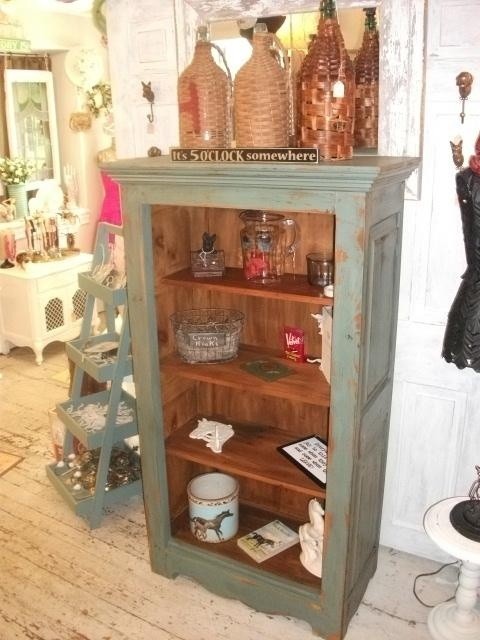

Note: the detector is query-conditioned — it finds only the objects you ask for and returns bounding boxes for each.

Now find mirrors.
[7,69,64,192]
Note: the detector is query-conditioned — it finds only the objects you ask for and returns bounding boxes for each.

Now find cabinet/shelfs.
[105,158,417,637]
[42,220,132,530]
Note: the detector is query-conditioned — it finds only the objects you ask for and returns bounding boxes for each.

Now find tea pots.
[236,212,299,286]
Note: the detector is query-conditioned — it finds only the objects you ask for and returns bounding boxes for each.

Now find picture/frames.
[278,435,339,488]
